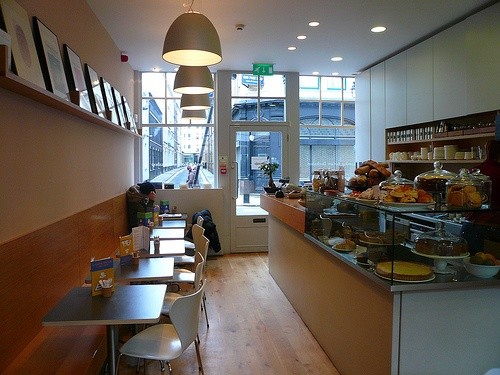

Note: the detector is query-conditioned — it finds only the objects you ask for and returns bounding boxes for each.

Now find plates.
[390,145,471,160]
[356,197,377,203]
[411,248,470,259]
[373,269,435,283]
[359,240,401,245]
[332,245,354,252]
[357,261,370,265]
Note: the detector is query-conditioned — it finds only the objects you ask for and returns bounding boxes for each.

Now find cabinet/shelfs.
[385,110,500,163]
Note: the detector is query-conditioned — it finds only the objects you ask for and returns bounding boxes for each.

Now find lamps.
[162,0,223,119]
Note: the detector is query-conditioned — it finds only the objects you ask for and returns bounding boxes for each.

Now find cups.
[103,286,112,297]
[132,257,139,264]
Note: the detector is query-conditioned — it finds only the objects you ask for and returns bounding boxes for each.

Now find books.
[131,225,150,251]
[145,207,159,225]
[91,256,115,296]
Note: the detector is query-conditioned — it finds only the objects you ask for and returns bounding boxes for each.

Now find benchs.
[0,194,130,375]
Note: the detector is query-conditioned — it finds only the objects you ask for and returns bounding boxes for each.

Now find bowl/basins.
[463,257,500,278]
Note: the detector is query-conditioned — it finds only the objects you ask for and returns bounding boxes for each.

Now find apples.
[470,252,498,266]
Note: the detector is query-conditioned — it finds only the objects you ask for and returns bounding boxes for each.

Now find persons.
[187,165,195,188]
[126,182,156,234]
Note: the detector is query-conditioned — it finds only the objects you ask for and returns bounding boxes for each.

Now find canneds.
[388,127,435,143]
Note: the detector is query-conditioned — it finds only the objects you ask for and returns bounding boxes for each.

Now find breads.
[348,159,391,186]
[335,242,356,250]
[380,185,433,204]
[357,188,378,200]
[447,185,483,208]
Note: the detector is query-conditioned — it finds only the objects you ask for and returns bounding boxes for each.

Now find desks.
[149,228,184,239]
[155,220,186,228]
[150,240,186,255]
[40,284,167,375]
[163,212,188,220]
[85,257,175,284]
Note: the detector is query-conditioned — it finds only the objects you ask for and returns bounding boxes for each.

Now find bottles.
[446,168,491,208]
[435,119,496,134]
[160,199,169,214]
[413,161,456,205]
[312,171,321,193]
[378,170,414,200]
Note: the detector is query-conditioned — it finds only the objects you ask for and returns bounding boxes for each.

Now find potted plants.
[260,163,280,193]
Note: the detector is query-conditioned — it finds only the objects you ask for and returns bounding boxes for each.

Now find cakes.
[414,232,468,256]
[359,231,405,244]
[375,262,432,280]
[415,175,456,191]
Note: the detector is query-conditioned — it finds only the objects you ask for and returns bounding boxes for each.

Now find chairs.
[116,211,210,375]
